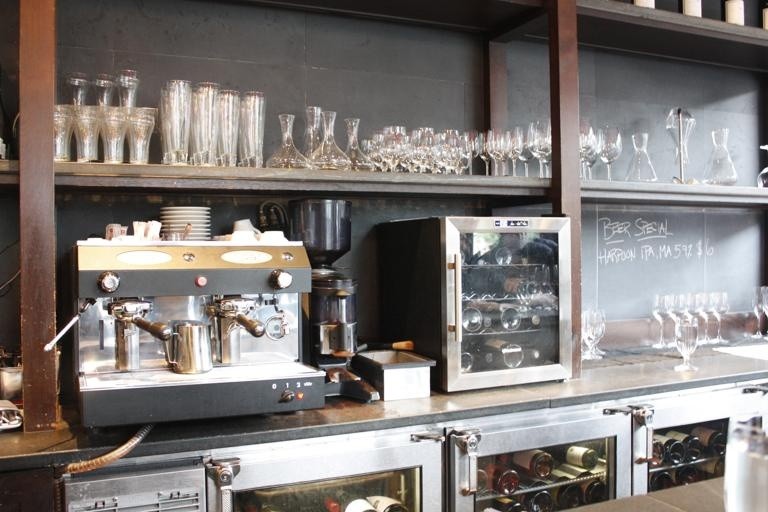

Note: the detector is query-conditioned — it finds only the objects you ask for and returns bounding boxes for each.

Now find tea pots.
[162,318,214,377]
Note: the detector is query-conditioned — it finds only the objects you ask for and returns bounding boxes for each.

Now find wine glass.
[579,306,609,362]
[362,120,623,182]
[651,286,767,372]
[756,145,768,187]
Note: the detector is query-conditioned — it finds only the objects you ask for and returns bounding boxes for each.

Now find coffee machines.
[288,198,360,401]
[71,243,324,433]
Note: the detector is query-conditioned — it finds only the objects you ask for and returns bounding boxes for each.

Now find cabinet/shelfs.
[1,1,768,437]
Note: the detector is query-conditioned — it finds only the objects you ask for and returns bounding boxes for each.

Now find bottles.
[663,107,699,183]
[245,488,414,512]
[623,131,659,184]
[460,236,558,374]
[265,104,372,170]
[473,430,728,510]
[704,128,735,185]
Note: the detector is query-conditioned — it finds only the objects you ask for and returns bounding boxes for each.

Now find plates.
[160,205,212,241]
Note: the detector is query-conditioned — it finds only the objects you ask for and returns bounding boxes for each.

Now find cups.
[53,71,157,165]
[678,0,702,17]
[105,223,128,241]
[632,0,655,9]
[724,0,744,25]
[158,79,264,167]
[723,412,766,512]
[230,220,287,242]
